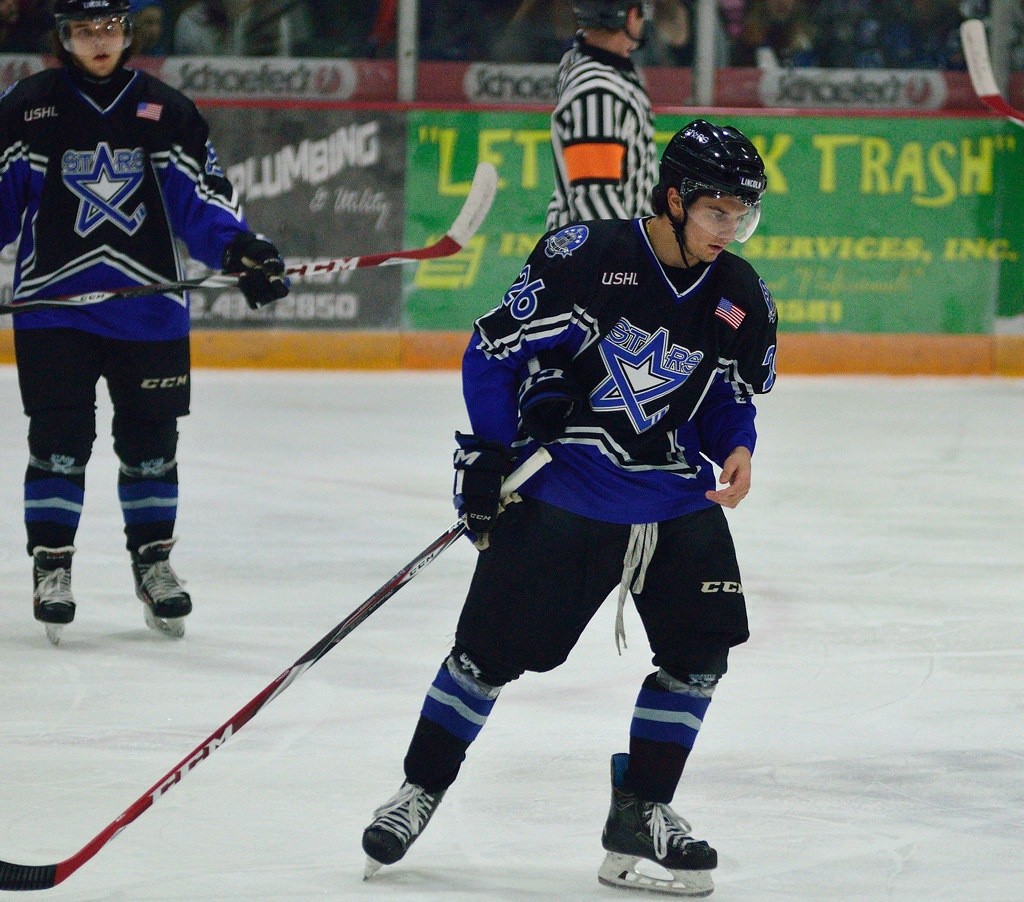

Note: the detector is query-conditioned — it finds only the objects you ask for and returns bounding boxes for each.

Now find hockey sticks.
[1,161,499,317]
[0,448,553,892]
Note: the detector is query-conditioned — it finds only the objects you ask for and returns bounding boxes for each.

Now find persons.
[0,0,1024,70]
[0,0,292,646]
[363,118,779,898]
[545,0,658,233]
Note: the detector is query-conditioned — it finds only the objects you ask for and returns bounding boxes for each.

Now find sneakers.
[598,754,717,896]
[361,778,447,881]
[33,546,76,647]
[129,537,192,638]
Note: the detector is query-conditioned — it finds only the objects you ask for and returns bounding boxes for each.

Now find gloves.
[223,233,291,309]
[451,430,525,559]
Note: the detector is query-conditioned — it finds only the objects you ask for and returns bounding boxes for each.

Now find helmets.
[50,0,131,23]
[573,0,645,32]
[661,118,769,203]
